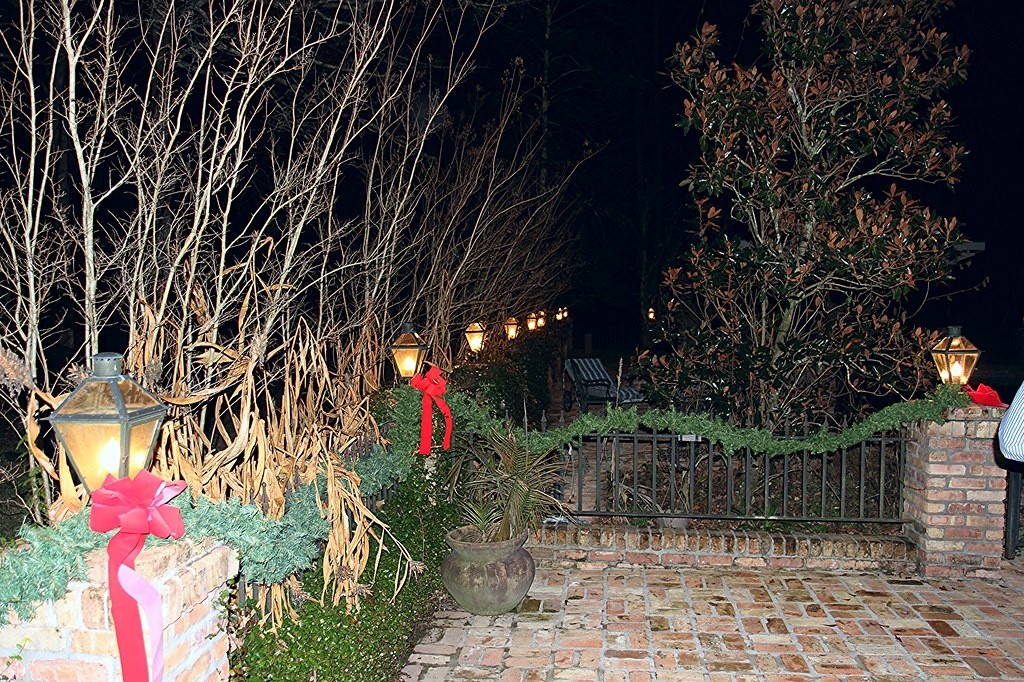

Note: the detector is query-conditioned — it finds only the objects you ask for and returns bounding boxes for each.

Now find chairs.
[562,358,646,414]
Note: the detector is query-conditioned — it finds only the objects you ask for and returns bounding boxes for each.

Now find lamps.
[39,353,174,495]
[930,326,986,386]
[526,306,569,331]
[464,322,488,359]
[388,323,430,385]
[504,316,521,342]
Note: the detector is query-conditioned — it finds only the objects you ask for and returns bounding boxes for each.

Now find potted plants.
[442,428,572,616]
